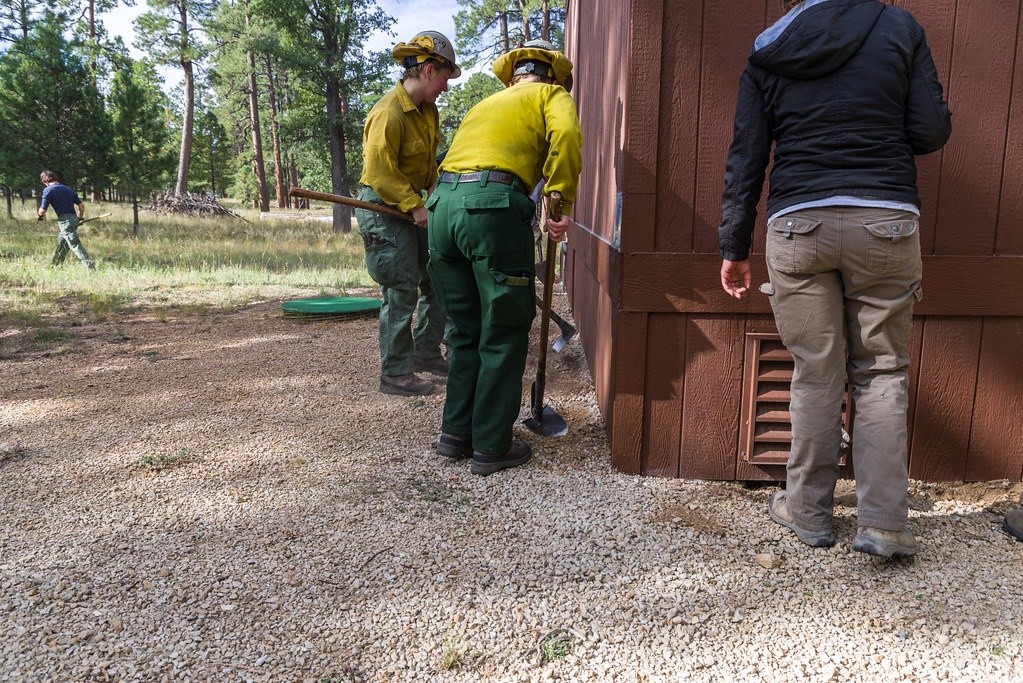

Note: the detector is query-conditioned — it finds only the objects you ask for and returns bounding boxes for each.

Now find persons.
[718,0,954,561]
[355,30,462,394]
[426,42,582,475]
[36,170,95,270]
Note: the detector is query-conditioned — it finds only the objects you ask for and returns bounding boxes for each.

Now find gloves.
[37,214,43,221]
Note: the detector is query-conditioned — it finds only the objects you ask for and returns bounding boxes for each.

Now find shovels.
[532,212,556,286]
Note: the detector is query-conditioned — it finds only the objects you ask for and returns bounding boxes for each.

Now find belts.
[440,172,528,195]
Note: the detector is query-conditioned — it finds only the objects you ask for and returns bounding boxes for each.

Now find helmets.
[494,39,573,93]
[393,30,461,78]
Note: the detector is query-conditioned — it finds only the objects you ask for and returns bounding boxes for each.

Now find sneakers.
[379,372,436,396]
[436,431,472,458]
[852,526,917,557]
[769,489,835,547]
[471,439,530,476]
[414,355,449,376]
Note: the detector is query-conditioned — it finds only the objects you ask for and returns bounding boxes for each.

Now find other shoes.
[1004,510,1023,540]
